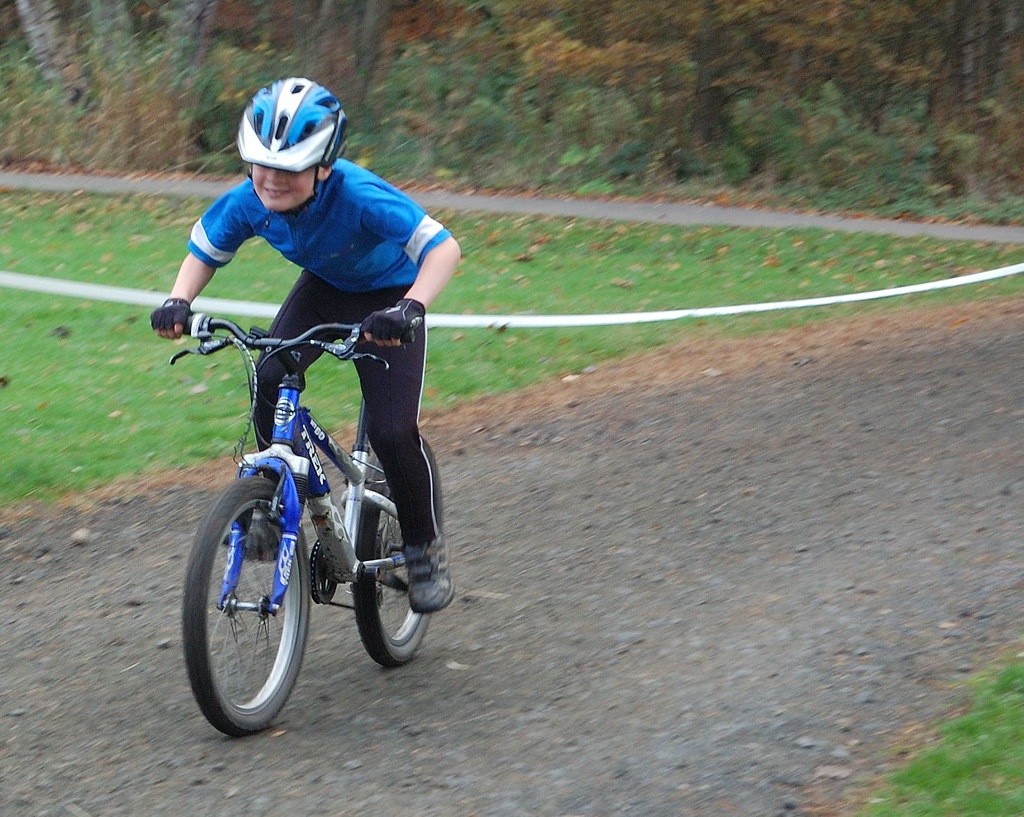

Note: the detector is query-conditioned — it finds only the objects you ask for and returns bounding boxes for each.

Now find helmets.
[237,78,347,173]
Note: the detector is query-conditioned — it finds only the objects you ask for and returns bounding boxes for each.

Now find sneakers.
[224,509,282,562]
[405,534,455,613]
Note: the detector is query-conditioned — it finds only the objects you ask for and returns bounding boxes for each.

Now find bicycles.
[167,311,433,741]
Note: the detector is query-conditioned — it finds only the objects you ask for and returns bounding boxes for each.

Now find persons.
[150,77,463,614]
